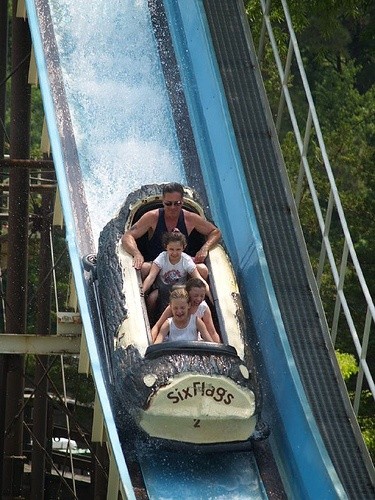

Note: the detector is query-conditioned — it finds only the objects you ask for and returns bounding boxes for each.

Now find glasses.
[162,200,183,208]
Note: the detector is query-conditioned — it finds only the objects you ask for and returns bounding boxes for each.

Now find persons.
[149,278,222,343]
[119,181,221,284]
[152,283,214,347]
[138,228,214,304]
